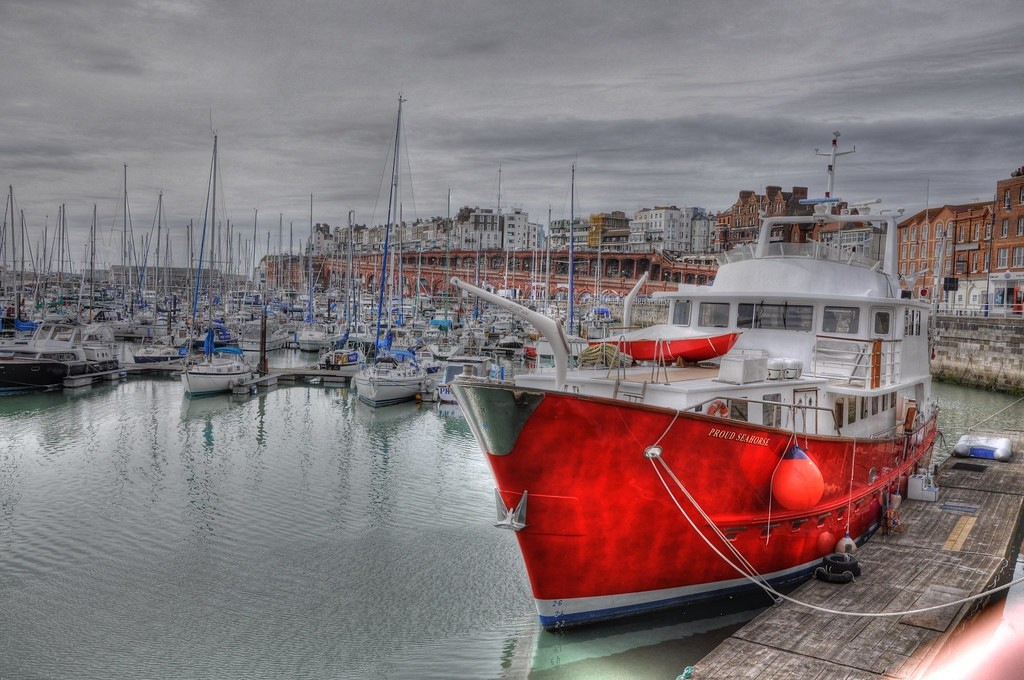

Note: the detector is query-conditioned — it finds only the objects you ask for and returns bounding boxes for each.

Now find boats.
[451,129,942,633]
[586,323,744,363]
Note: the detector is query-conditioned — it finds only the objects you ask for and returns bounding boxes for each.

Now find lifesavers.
[707,400,729,418]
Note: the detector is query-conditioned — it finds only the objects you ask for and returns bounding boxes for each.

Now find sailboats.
[0,92,620,409]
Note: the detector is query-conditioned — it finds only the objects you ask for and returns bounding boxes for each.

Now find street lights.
[983,193,1013,317]
[943,213,958,278]
